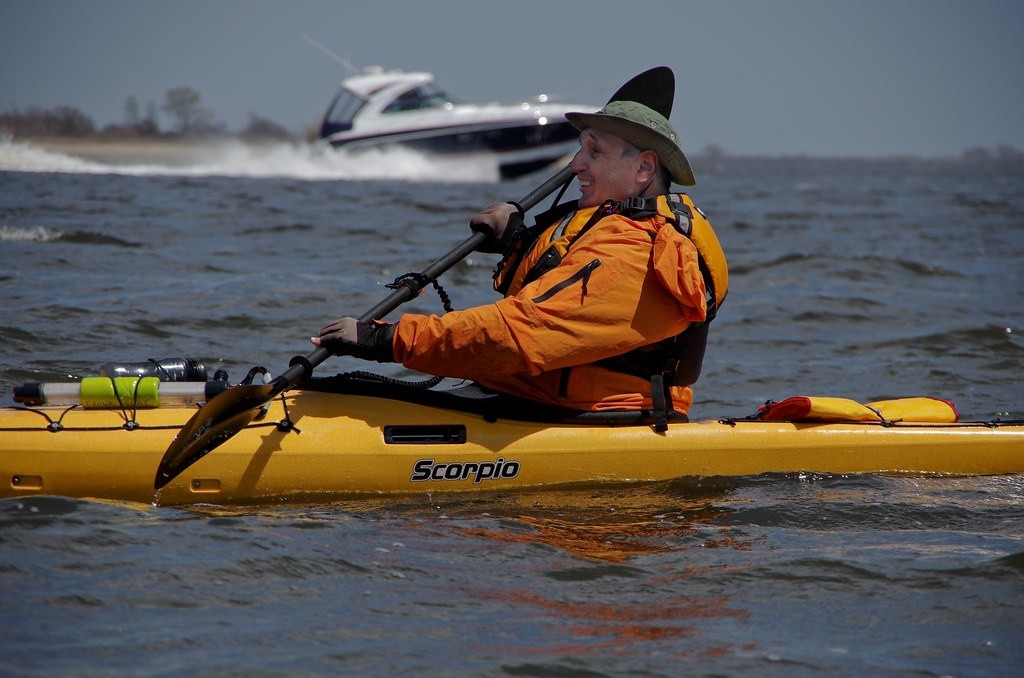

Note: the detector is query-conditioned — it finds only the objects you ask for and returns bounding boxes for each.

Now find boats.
[0,375,1024,497]
[309,69,602,182]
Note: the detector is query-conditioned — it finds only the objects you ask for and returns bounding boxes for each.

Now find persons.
[310,101,729,425]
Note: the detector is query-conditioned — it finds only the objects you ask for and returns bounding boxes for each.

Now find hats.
[564,66,697,186]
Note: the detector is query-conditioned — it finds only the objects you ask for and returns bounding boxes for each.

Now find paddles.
[155,161,579,494]
